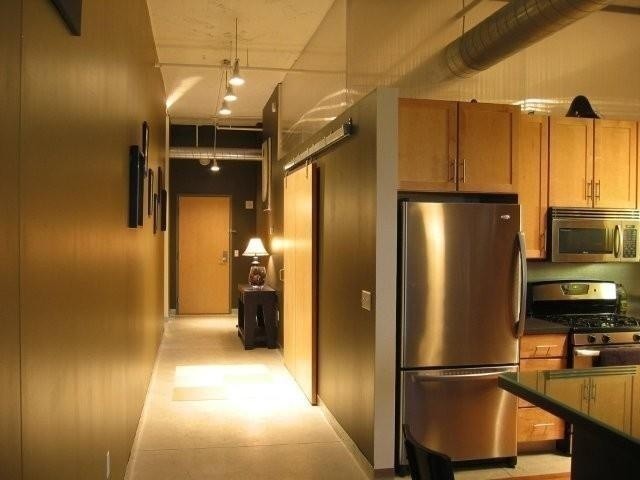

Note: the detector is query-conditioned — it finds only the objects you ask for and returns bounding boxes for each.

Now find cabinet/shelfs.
[516,332,568,455]
[548,116,636,212]
[396,96,521,194]
[237,283,277,351]
[517,112,549,261]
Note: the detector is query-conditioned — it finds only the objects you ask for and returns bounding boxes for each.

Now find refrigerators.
[397,189,529,476]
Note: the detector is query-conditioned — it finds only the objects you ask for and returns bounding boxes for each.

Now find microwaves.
[550,217,639,263]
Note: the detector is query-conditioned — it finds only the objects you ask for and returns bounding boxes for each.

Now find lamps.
[210,120,219,172]
[216,17,245,116]
[241,238,269,288]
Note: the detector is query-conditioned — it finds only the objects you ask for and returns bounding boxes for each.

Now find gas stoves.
[538,311,640,345]
